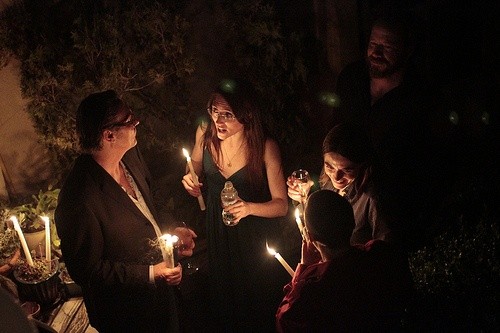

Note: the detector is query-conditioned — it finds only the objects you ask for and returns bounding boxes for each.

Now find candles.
[155,233,180,269]
[265,242,294,280]
[290,208,311,241]
[179,148,211,211]
[40,213,51,263]
[11,214,38,267]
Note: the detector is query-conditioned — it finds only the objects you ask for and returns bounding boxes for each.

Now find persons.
[332,17,452,247]
[275,191,424,333]
[54,88,197,333]
[287,123,400,252]
[182,87,301,310]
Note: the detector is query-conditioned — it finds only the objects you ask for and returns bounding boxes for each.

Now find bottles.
[220,180,240,227]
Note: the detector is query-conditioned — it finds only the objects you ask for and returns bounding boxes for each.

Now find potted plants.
[59,266,84,296]
[0,227,20,278]
[11,204,48,258]
[13,252,62,309]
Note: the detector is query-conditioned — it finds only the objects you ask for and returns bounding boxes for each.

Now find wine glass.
[168,221,201,275]
[291,169,311,227]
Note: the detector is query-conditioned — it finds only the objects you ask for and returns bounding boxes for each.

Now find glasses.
[103,112,138,129]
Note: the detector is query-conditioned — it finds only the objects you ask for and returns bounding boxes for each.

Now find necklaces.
[223,141,243,167]
